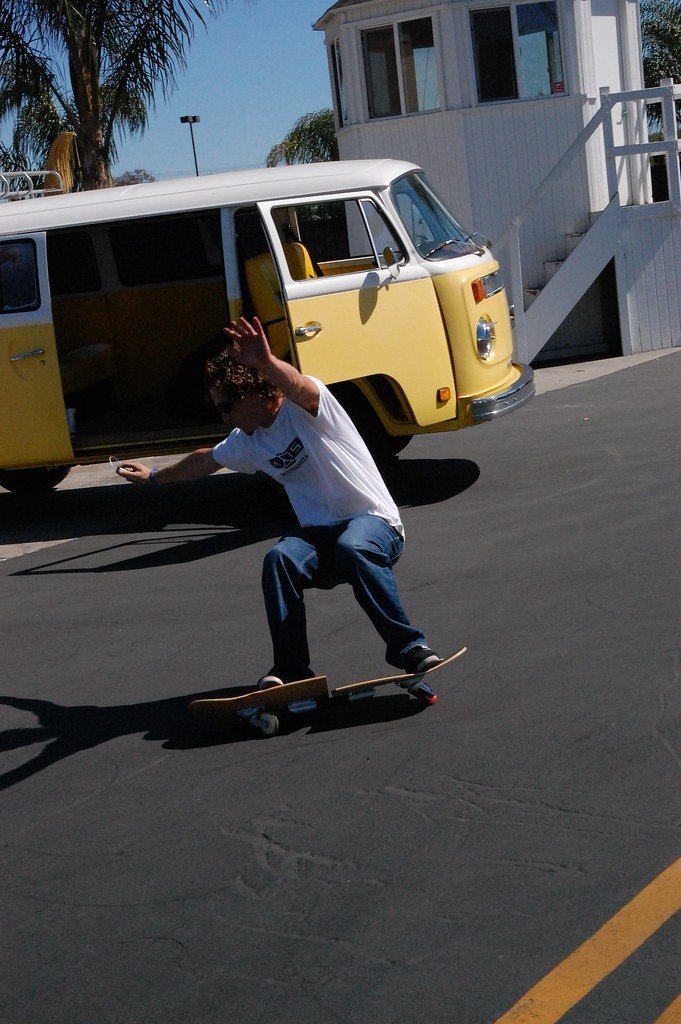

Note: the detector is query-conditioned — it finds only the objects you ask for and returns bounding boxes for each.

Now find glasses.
[216,397,236,414]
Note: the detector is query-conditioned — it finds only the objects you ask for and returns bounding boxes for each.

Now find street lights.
[179,114,200,176]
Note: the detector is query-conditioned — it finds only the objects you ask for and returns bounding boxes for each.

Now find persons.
[116,316,442,689]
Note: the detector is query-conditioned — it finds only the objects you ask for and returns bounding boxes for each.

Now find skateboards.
[186,644,468,737]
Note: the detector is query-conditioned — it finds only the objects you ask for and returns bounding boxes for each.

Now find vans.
[1,166,537,499]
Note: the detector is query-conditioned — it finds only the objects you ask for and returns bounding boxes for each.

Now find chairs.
[58,344,115,425]
[245,243,319,361]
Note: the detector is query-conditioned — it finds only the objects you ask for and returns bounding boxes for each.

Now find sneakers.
[386,646,440,673]
[261,666,303,688]
